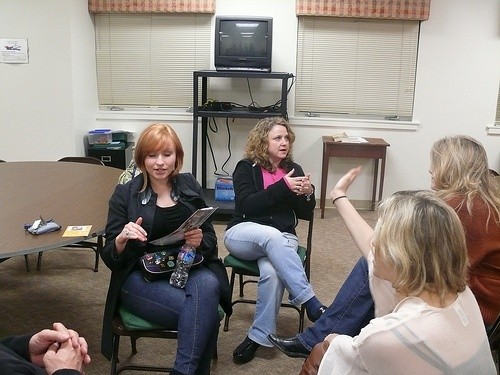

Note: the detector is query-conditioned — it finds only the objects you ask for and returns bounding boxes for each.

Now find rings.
[126,232,130,236]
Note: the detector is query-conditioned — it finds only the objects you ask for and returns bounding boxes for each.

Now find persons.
[297,165,497,375]
[268,136,500,358]
[224,116,329,365]
[101,124,233,370]
[0,323,92,375]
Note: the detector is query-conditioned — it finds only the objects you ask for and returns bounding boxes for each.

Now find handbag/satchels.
[140,246,204,282]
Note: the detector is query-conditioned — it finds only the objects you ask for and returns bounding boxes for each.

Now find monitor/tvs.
[214,16,273,73]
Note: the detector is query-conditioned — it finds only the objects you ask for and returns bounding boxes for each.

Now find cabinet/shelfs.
[192,71,293,225]
[88,143,134,171]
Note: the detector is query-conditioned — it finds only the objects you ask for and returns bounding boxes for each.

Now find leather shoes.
[233,336,260,364]
[268,333,311,357]
[306,305,327,322]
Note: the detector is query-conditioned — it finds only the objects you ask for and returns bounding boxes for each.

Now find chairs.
[0,157,106,272]
[111,305,225,375]
[220,184,315,333]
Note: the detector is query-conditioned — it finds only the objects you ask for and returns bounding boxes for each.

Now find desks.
[0,161,125,257]
[320,136,390,219]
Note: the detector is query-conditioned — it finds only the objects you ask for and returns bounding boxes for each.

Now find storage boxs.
[215,176,235,202]
[89,130,110,145]
[113,133,127,142]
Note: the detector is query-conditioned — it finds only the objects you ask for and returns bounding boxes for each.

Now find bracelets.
[308,185,314,196]
[332,196,347,205]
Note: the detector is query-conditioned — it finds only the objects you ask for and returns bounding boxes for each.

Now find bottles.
[169,245,196,289]
